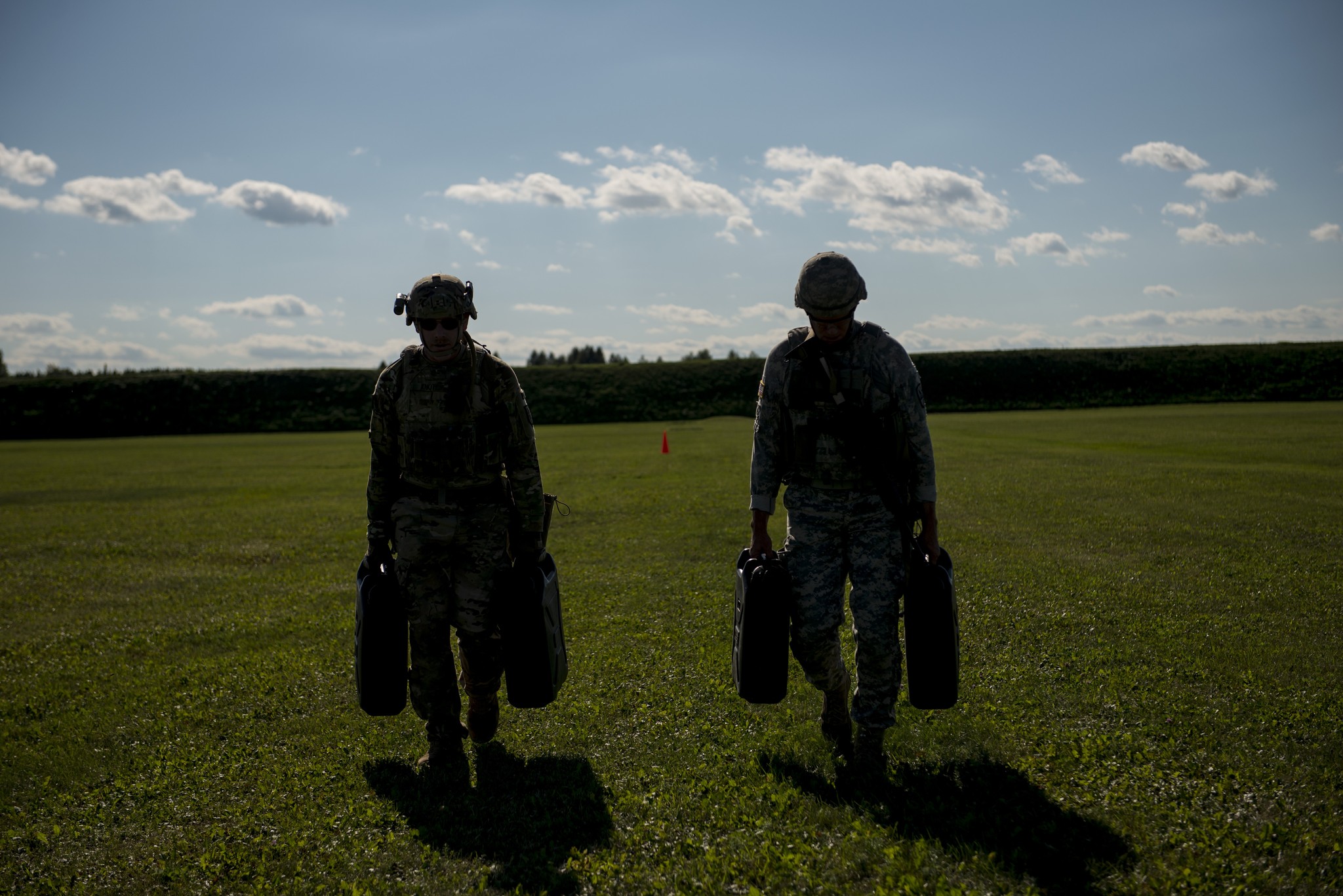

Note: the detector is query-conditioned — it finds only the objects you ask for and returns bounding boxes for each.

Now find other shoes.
[418,740,463,769]
[854,726,884,768]
[820,672,853,744]
[467,693,500,743]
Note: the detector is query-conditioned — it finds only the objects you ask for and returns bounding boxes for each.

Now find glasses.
[417,315,460,331]
[809,310,854,327]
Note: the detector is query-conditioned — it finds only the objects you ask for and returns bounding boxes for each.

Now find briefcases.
[730,549,793,704]
[355,558,407,716]
[504,552,569,708]
[904,547,960,709]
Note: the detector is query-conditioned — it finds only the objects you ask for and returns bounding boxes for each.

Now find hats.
[795,252,867,316]
[393,273,478,326]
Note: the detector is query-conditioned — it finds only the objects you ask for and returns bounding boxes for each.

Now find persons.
[731,252,945,791]
[362,272,553,782]
